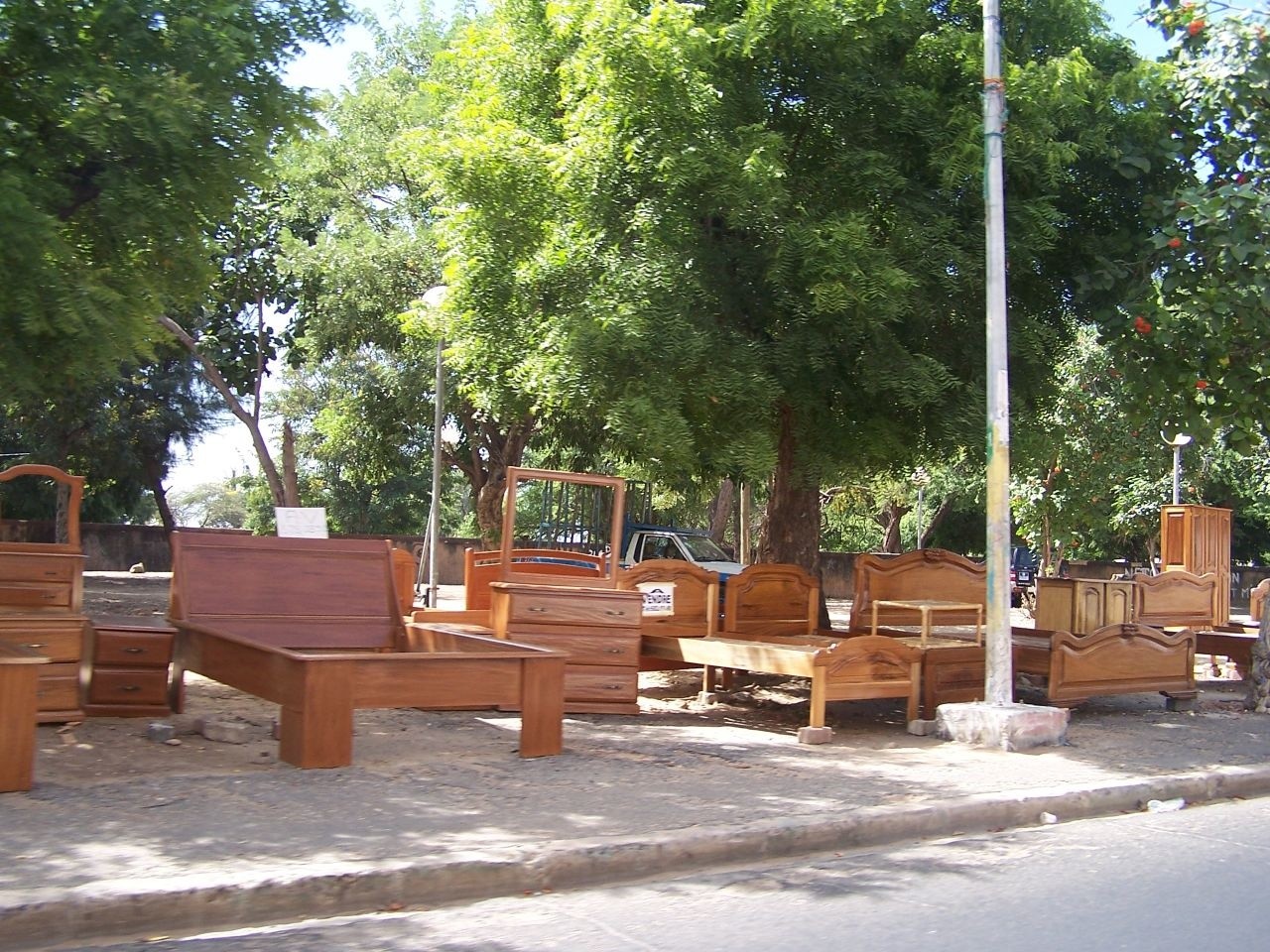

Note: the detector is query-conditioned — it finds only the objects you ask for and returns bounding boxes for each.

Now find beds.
[164,527,574,769]
[720,564,991,726]
[409,544,605,614]
[846,546,1197,711]
[1125,571,1257,684]
[614,559,929,733]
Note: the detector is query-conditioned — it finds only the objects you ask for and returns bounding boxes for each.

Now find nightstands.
[80,613,178,720]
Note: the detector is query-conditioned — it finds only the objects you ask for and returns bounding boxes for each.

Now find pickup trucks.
[524,525,753,605]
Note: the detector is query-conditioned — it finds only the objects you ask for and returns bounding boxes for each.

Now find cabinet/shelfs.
[1033,573,1137,640]
[487,465,645,716]
[0,464,88,729]
[1159,502,1234,631]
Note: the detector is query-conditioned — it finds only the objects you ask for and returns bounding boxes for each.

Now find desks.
[0,638,54,793]
[870,596,984,650]
